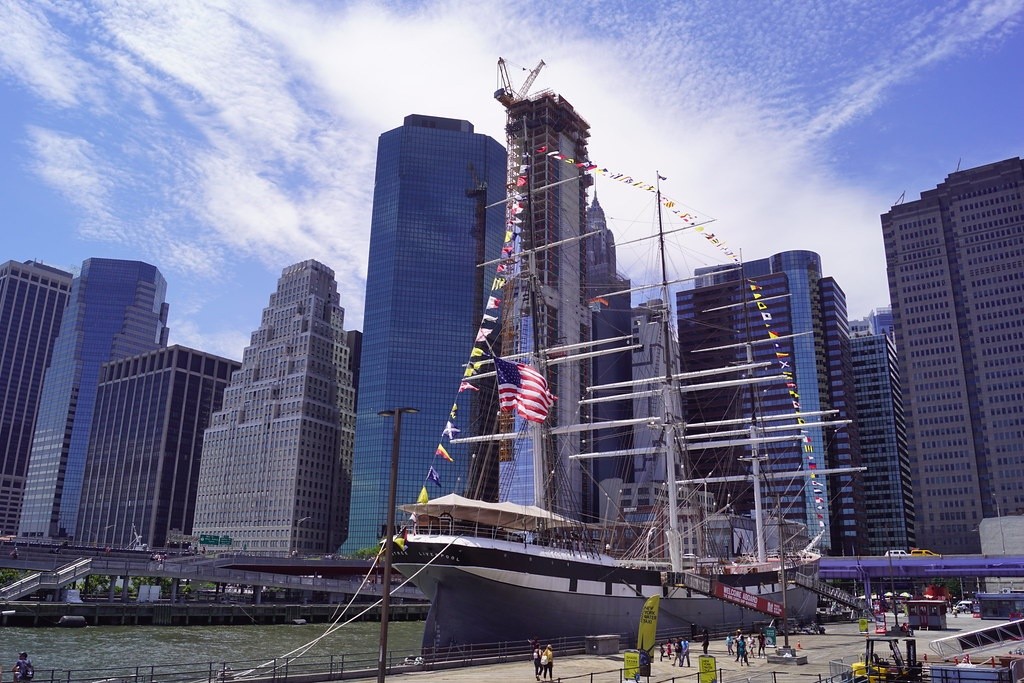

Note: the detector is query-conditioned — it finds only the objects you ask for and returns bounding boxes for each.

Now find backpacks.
[22,659,34,681]
[541,656,548,665]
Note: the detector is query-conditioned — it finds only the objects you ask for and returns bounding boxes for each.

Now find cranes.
[492,56,548,110]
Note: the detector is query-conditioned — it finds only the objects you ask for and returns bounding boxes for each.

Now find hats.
[547,645,552,649]
[736,629,742,633]
[18,652,27,658]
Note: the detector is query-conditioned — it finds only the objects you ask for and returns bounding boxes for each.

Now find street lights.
[295,517,313,557]
[376,407,421,683]
[993,493,1005,554]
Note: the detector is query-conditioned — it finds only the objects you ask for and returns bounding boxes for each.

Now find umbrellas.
[884,591,893,596]
[900,591,913,597]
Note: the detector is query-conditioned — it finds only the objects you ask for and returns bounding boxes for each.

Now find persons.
[680,636,691,667]
[666,639,674,660]
[542,645,555,681]
[11,652,33,683]
[528,636,538,661]
[659,643,668,662]
[735,629,750,666]
[671,637,681,666]
[757,631,766,659]
[702,628,709,655]
[747,633,756,657]
[725,631,735,655]
[533,643,543,681]
[953,605,957,617]
[962,603,966,613]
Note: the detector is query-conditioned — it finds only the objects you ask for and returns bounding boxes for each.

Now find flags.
[393,166,530,549]
[537,144,827,530]
[493,355,559,423]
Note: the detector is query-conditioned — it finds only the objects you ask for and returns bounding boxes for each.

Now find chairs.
[873,654,889,666]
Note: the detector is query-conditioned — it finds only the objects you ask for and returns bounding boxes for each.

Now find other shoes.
[536,675,540,681]
[672,663,676,666]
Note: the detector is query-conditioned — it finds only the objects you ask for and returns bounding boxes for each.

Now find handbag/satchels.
[751,643,756,648]
[534,653,538,658]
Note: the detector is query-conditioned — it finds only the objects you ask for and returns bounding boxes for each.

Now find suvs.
[885,550,911,557]
[910,550,940,556]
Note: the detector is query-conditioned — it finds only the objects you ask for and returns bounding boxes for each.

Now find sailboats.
[384,105,869,676]
